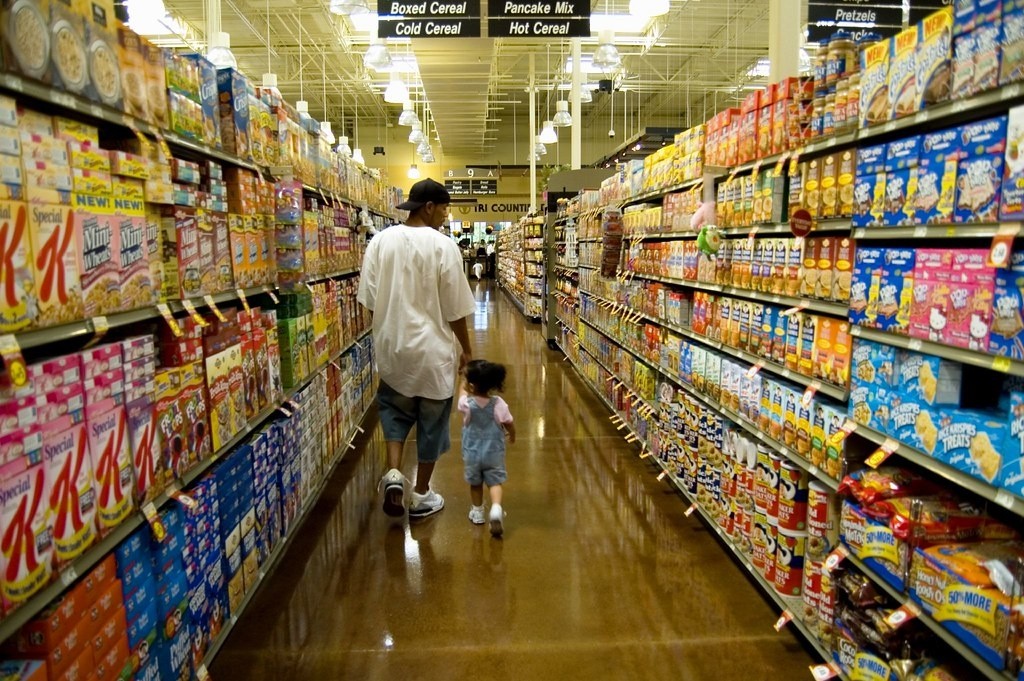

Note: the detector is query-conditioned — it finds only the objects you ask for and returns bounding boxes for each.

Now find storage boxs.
[580,0,1023,681]
[0,0,381,680]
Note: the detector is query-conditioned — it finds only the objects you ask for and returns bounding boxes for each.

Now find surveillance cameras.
[608,130,615,138]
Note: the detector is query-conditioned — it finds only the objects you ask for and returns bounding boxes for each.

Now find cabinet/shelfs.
[0,75,408,681]
[495,222,543,324]
[548,79,1023,681]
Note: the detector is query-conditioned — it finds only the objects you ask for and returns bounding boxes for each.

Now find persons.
[356,178,477,519]
[457,359,516,536]
[446,231,497,281]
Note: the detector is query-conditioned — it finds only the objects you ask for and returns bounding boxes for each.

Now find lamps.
[120,0,670,176]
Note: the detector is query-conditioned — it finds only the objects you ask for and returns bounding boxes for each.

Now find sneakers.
[378,469,410,517]
[469,508,486,524]
[409,485,444,518]
[488,506,507,535]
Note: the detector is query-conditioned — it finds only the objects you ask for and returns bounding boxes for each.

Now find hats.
[396,178,451,210]
